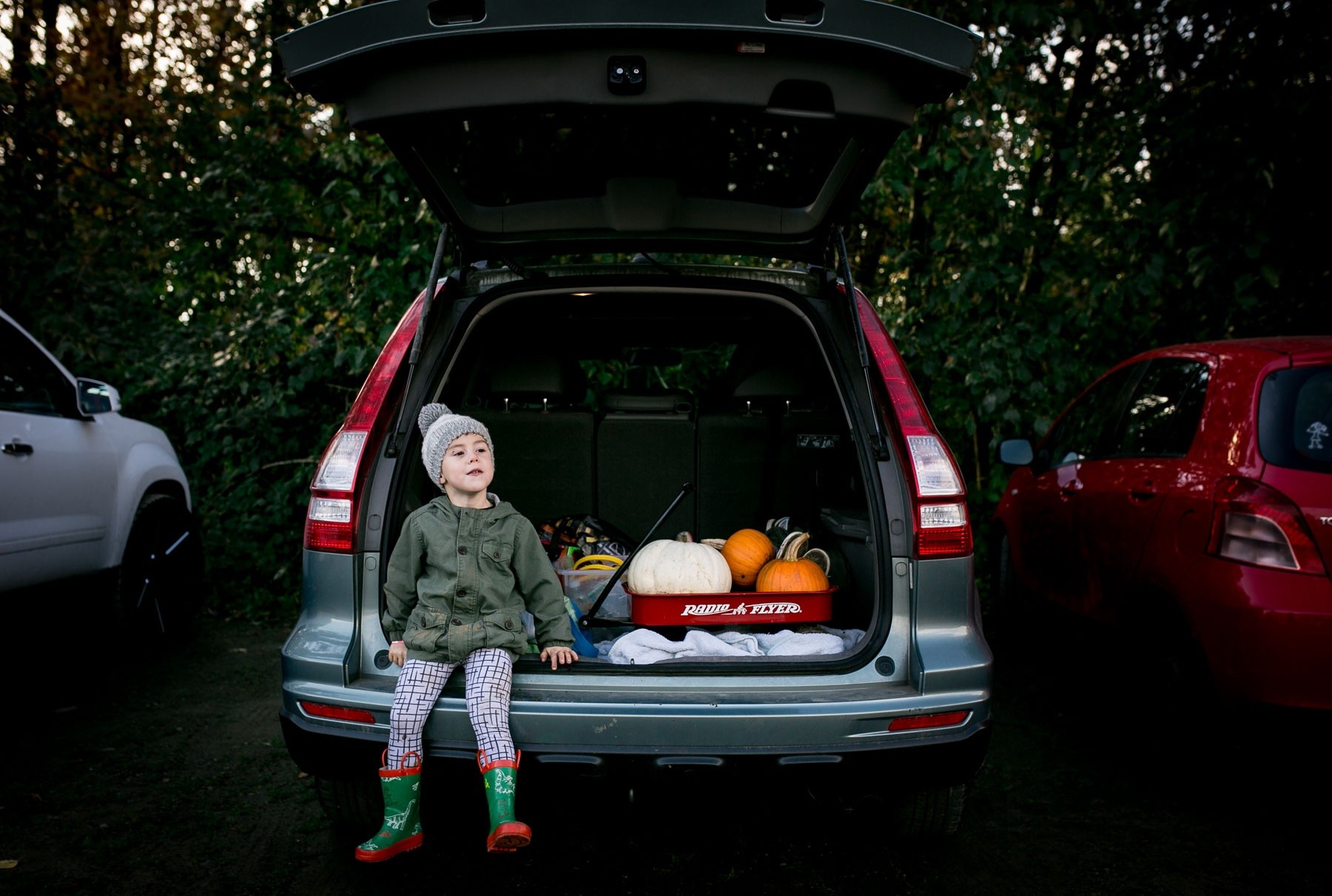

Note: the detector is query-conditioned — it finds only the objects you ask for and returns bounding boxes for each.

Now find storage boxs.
[553,560,632,623]
[625,584,835,626]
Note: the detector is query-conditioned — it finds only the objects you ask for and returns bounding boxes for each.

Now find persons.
[355,403,579,859]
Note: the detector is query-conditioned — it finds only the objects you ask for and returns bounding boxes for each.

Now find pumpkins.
[628,529,830,592]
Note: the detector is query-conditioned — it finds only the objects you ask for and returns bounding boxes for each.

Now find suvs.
[271,0,990,834]
[0,310,199,643]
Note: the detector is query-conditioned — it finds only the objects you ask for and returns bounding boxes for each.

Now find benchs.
[462,365,814,552]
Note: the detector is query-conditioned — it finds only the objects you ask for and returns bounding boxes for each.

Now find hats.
[418,404,494,492]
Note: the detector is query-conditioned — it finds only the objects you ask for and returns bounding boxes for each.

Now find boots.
[356,749,422,862]
[477,750,532,851]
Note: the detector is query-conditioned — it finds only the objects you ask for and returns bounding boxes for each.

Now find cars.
[994,333,1332,791]
